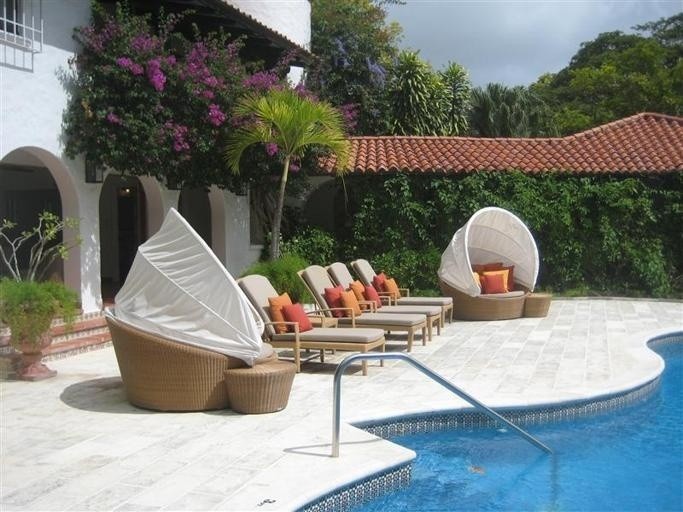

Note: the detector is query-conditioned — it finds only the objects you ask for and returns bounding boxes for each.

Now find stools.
[524,293,552,318]
[223,359,297,413]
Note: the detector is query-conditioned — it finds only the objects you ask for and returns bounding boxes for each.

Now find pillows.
[473,262,515,294]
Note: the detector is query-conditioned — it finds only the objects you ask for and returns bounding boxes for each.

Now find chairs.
[236,257,455,375]
[102,207,265,412]
[436,205,539,321]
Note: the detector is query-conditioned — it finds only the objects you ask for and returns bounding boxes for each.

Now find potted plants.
[0,212,84,382]
